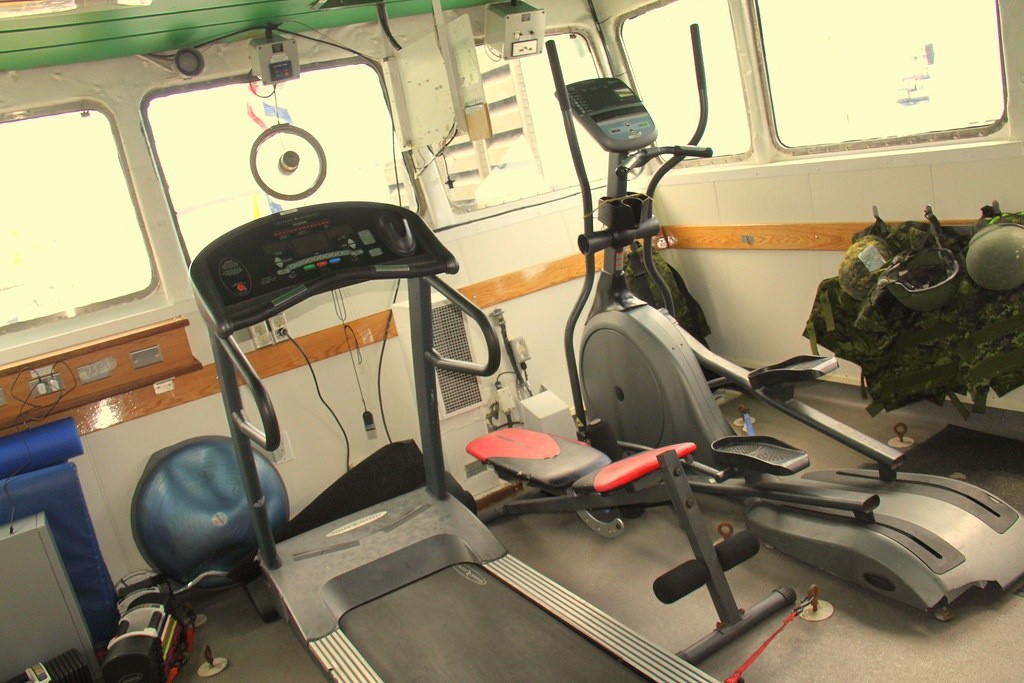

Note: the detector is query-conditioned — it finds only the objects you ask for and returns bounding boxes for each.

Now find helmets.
[965,222,1024,291]
[878,246,959,312]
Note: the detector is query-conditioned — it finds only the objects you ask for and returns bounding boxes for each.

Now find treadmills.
[186,199,723,683]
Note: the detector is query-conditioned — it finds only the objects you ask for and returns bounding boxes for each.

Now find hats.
[838,234,896,303]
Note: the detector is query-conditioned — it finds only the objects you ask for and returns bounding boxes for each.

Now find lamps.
[172,48,204,80]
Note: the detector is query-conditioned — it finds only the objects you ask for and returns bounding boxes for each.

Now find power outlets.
[248,313,291,350]
[28,372,66,399]
